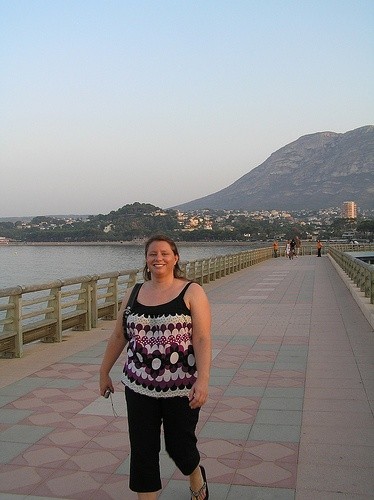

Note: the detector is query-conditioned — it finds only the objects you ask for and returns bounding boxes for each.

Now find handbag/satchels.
[122,282,143,340]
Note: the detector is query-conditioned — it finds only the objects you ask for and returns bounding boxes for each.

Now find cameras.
[104,388,111,398]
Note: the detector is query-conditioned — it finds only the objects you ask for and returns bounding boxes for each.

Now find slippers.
[189,465,209,500]
